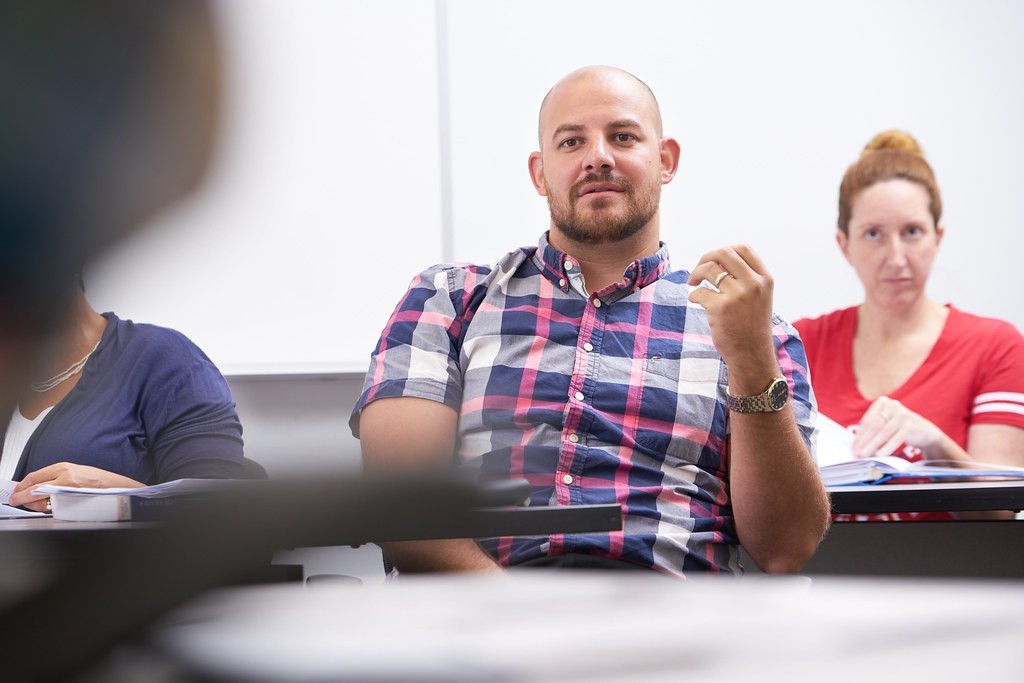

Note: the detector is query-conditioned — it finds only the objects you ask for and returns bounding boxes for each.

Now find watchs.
[725,375,790,414]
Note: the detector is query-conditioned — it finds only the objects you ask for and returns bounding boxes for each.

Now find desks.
[0,502,622,554]
[737,480,1024,580]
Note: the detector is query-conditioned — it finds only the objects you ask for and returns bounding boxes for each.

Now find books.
[817,456,1024,486]
[50,494,239,521]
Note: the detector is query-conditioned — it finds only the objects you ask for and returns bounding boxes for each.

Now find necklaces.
[27,338,100,392]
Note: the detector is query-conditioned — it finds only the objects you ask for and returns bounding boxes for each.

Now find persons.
[0,263,247,514]
[350,66,830,576]
[790,130,1023,481]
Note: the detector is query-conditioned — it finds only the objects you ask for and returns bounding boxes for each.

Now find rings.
[879,411,888,421]
[715,271,729,288]
[47,498,52,510]
